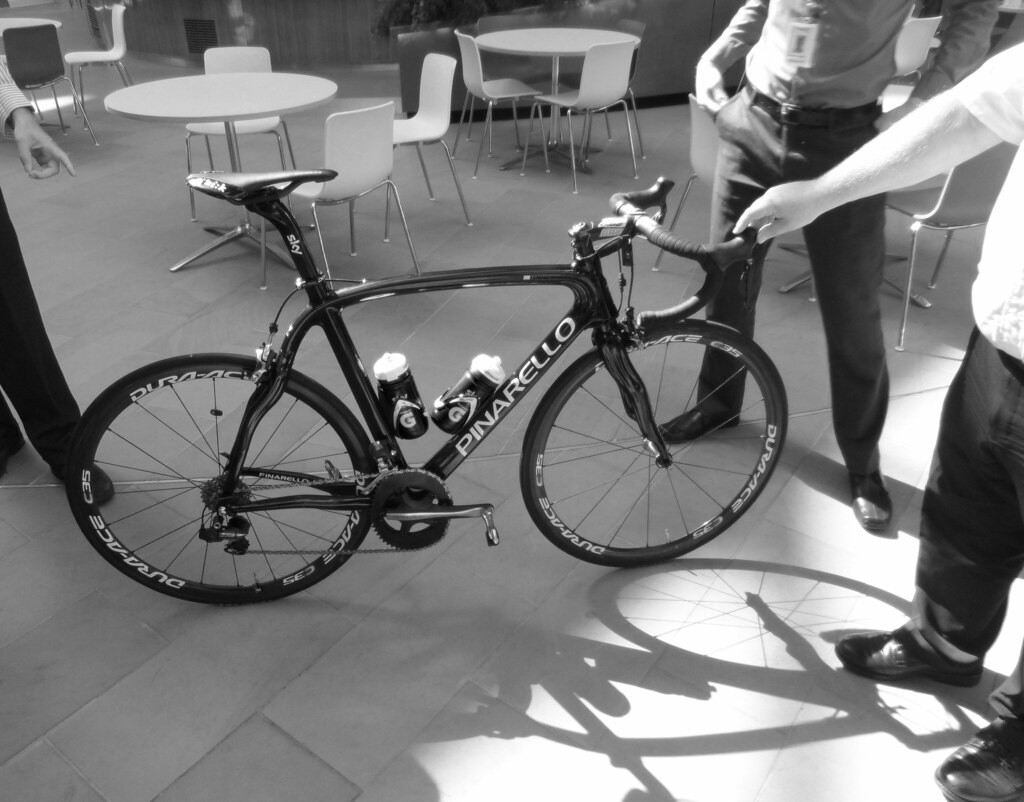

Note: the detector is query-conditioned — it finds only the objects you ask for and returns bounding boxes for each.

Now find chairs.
[261,101,421,312]
[64,4,134,131]
[520,19,647,195]
[354,53,473,243]
[652,93,768,273]
[883,141,1019,352]
[4,25,100,147]
[185,47,297,221]
[454,28,551,179]
[891,15,943,84]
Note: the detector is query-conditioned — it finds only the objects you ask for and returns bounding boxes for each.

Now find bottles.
[431,354,505,435]
[373,352,429,440]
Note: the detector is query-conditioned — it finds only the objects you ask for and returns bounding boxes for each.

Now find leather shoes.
[52,461,114,506]
[850,469,892,530]
[935,716,1024,802]
[834,624,984,688]
[658,408,741,444]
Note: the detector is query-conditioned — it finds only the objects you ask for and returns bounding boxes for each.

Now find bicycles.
[66,167,792,604]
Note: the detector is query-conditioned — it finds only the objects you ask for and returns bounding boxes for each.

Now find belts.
[746,79,882,128]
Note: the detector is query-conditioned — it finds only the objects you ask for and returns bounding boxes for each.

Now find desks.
[0,18,62,36]
[476,25,640,174]
[775,84,932,308]
[105,72,338,273]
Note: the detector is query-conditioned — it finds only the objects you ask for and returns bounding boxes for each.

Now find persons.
[0,58,115,506]
[732,40,1024,802]
[656,0,1001,530]
